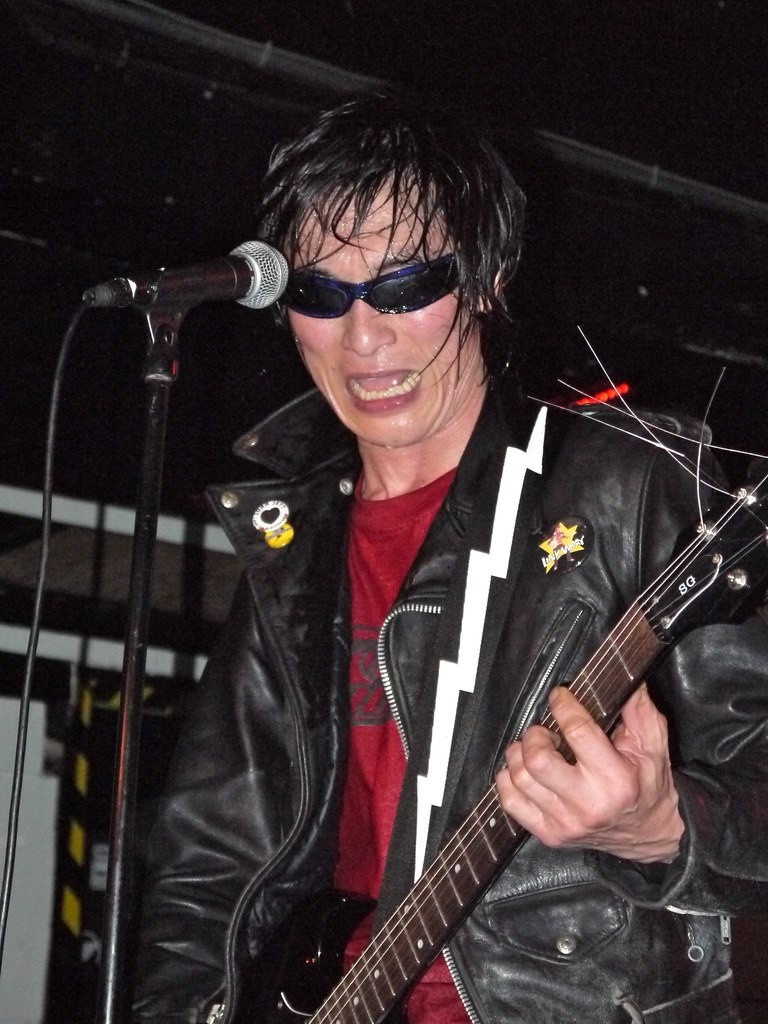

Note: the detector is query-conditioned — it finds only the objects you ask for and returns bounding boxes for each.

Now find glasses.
[278,252,461,320]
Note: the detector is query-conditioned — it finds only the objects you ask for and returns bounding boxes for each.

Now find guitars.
[207,468,767,1023]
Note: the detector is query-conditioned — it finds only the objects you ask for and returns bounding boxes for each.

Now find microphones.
[83,239,292,312]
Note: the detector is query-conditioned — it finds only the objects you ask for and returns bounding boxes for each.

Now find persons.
[110,93,768,1023]
